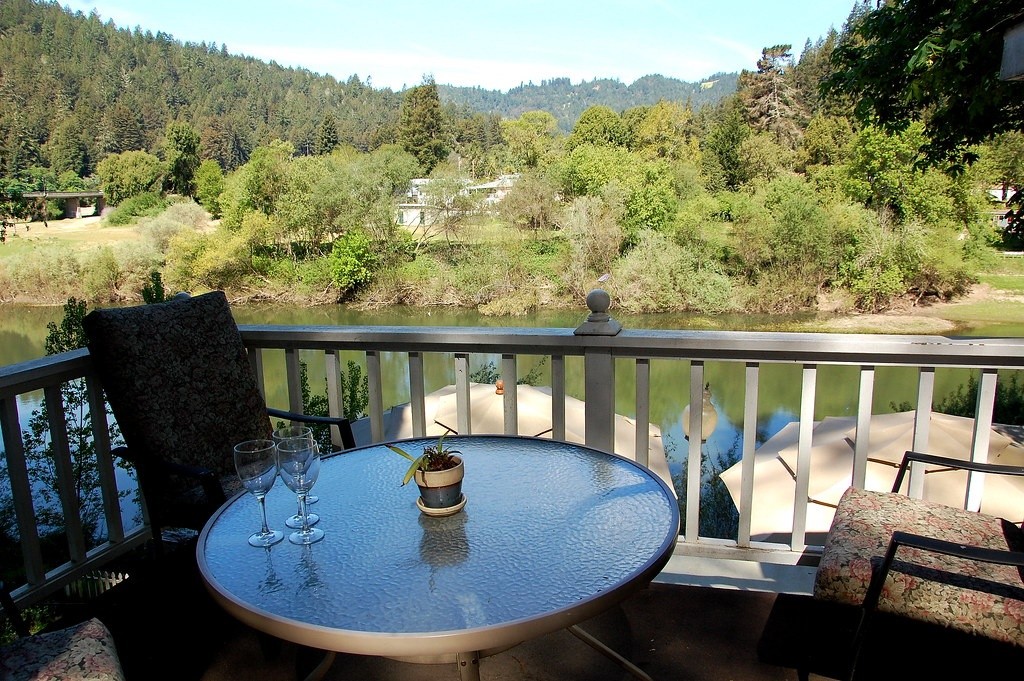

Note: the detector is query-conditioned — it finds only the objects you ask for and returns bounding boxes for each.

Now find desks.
[204,431,679,681]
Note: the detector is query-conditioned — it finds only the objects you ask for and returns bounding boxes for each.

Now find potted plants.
[382,428,467,517]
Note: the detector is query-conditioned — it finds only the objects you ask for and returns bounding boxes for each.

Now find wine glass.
[281,434,319,505]
[234,440,284,547]
[272,427,319,528]
[277,437,325,545]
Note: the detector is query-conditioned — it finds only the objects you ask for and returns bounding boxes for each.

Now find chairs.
[79,289,357,602]
[796,417,1024,681]
